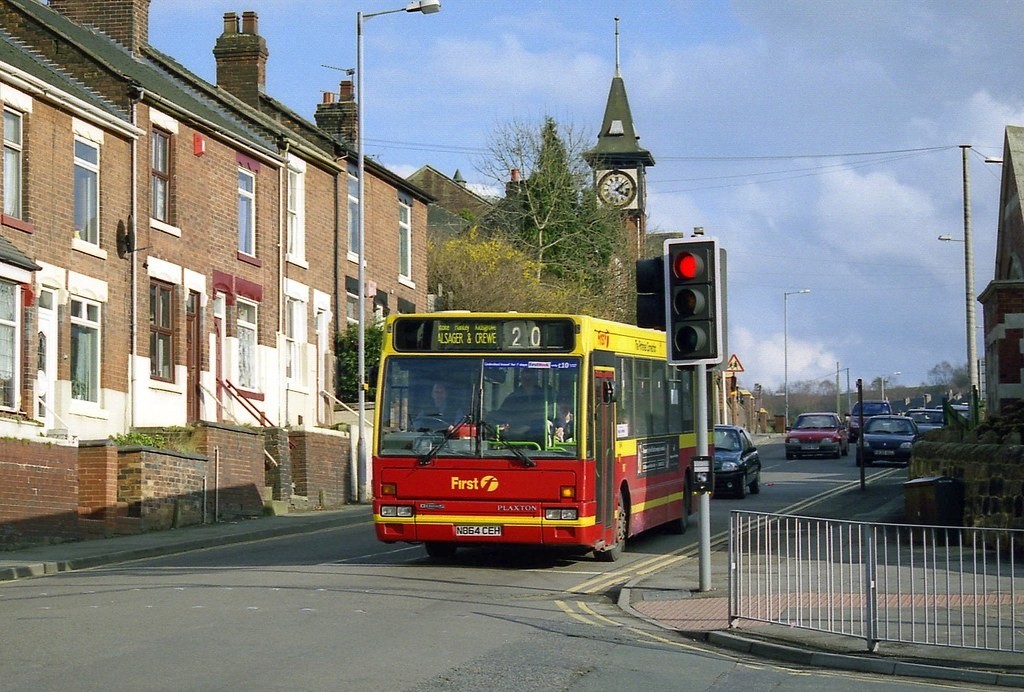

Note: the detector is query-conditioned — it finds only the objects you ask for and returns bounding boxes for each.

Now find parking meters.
[689,456,716,496]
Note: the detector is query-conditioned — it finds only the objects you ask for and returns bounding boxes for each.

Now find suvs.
[845,400,894,443]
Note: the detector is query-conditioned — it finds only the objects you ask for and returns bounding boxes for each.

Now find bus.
[372,308,716,562]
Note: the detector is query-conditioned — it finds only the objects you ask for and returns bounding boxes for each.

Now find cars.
[785,411,849,458]
[856,415,920,468]
[936,405,969,425]
[712,426,763,501]
[903,408,945,434]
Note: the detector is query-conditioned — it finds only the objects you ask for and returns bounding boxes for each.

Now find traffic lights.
[636,257,666,331]
[665,237,722,366]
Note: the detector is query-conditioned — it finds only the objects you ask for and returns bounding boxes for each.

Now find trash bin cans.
[901,475,964,546]
[773,414,786,433]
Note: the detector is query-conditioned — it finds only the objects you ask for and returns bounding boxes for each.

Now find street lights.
[783,290,811,434]
[938,235,979,404]
[881,372,902,402]
[357,1,444,504]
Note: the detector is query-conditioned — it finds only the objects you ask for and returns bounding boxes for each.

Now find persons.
[485,367,574,451]
[900,424,908,431]
[406,382,463,440]
[722,434,736,448]
[617,413,631,436]
